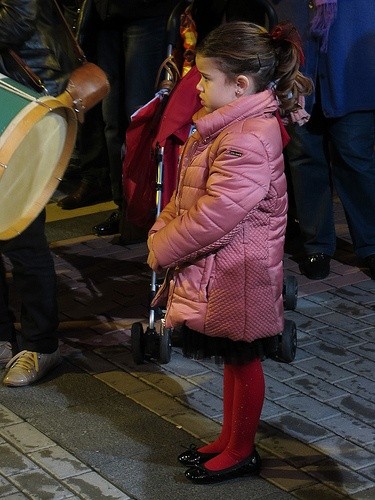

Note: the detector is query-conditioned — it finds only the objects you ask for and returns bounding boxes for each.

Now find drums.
[0,70,78,241]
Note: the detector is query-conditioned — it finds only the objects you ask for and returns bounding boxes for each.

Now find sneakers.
[0,341,18,365]
[1,347,60,384]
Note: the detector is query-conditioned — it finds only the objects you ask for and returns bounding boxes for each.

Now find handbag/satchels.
[44,63,110,122]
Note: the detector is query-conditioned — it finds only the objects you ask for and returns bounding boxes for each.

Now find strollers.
[131,0,297,363]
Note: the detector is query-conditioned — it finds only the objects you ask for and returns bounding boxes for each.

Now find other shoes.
[301,252,330,279]
[92,212,121,236]
[56,182,99,209]
[177,441,262,484]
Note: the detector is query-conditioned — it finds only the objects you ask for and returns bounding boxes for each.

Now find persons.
[147,21,315,483]
[270,0,375,279]
[58,0,177,236]
[0,0,63,386]
